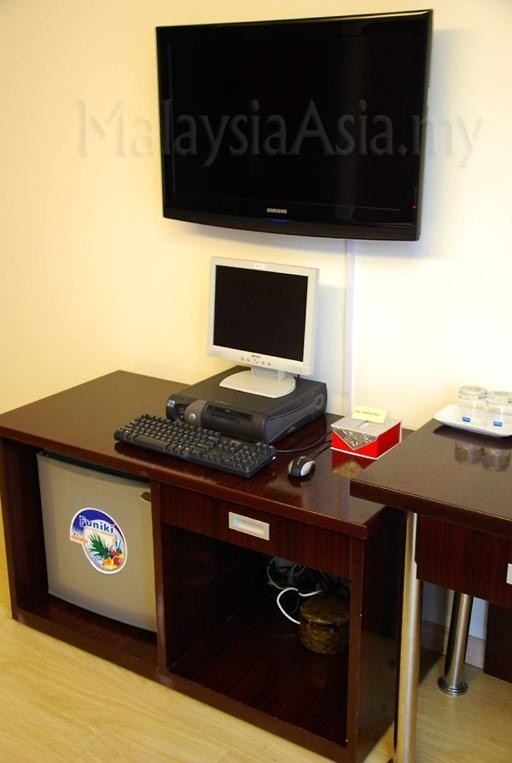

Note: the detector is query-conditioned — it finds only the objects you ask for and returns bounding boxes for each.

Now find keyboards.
[114,414,278,479]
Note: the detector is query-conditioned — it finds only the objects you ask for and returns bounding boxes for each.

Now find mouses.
[287,454,315,478]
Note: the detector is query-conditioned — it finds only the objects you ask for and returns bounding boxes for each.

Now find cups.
[456,386,485,426]
[485,394,510,432]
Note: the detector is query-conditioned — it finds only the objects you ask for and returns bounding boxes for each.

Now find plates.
[433,403,511,439]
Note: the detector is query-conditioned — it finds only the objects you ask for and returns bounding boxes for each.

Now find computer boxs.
[166,365,327,444]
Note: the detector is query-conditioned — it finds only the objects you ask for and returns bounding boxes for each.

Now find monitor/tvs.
[206,256,319,399]
[155,9,433,241]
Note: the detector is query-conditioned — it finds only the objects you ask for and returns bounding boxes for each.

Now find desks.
[349,417,512,696]
[0,370,447,763]
